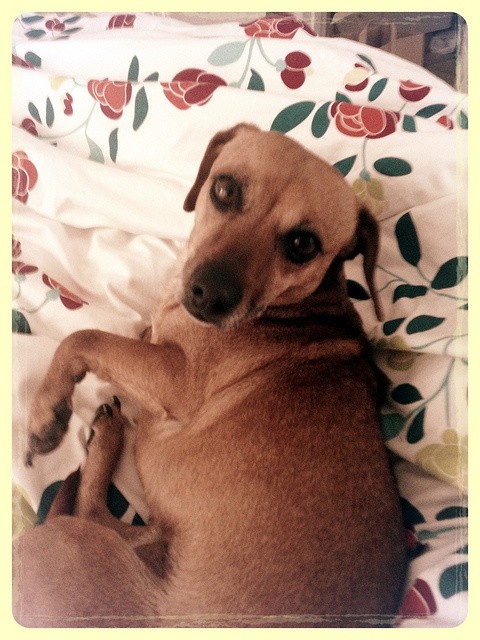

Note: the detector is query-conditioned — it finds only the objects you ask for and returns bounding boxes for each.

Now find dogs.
[12,124,407,629]
